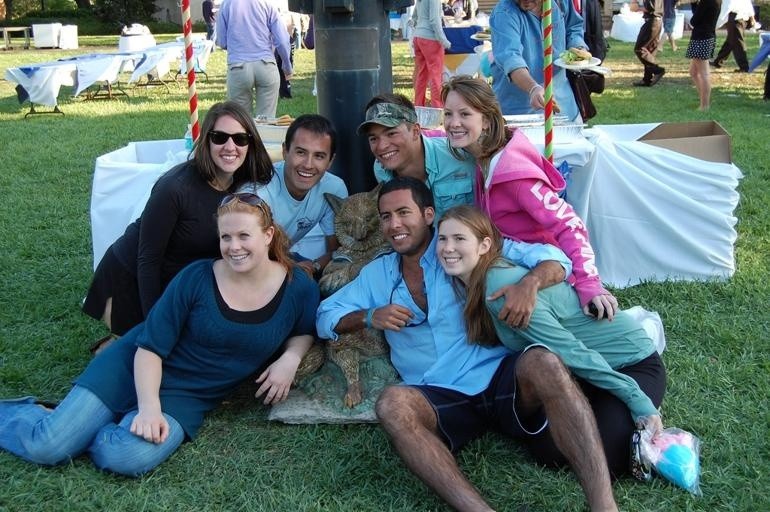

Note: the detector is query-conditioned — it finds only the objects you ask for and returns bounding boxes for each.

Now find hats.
[354,102,419,138]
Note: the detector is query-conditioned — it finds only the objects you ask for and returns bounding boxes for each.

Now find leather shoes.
[632,79,651,87]
[650,68,665,87]
[709,60,721,68]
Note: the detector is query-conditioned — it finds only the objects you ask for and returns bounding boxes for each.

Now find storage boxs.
[636,119,733,165]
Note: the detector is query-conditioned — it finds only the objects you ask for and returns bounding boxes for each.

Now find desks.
[5,39,214,120]
[442,24,484,54]
[0,25,32,49]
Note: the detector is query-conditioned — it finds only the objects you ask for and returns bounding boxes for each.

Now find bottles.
[185,123,193,152]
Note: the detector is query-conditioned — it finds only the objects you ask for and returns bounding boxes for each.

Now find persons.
[234,114,350,283]
[443,70,623,326]
[399,0,770,124]
[315,174,621,512]
[203,0,318,122]
[435,204,667,484]
[81,98,277,358]
[2,189,322,480]
[362,91,482,230]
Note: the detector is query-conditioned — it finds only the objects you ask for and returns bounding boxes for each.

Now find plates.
[555,57,602,70]
[474,44,492,54]
[471,33,492,42]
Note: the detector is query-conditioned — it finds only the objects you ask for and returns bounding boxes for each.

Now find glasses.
[220,192,270,227]
[209,129,253,147]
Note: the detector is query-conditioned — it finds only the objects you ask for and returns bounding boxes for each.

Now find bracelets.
[366,306,375,330]
[310,259,322,277]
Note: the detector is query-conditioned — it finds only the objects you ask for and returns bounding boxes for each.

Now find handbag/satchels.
[568,71,597,124]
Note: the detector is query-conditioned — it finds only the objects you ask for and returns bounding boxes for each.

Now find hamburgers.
[559,47,593,67]
